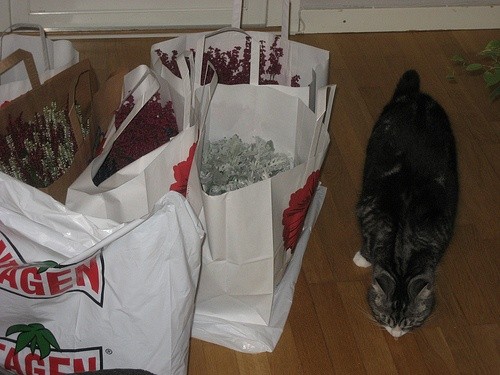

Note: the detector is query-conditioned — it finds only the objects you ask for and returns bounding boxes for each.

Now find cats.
[352,69,459,338]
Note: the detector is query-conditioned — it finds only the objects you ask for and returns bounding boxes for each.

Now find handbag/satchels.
[0,1,339,374]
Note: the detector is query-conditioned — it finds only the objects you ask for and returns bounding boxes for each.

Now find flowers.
[197,133,294,195]
[0,97,90,187]
[93,90,179,184]
[156,33,302,88]
